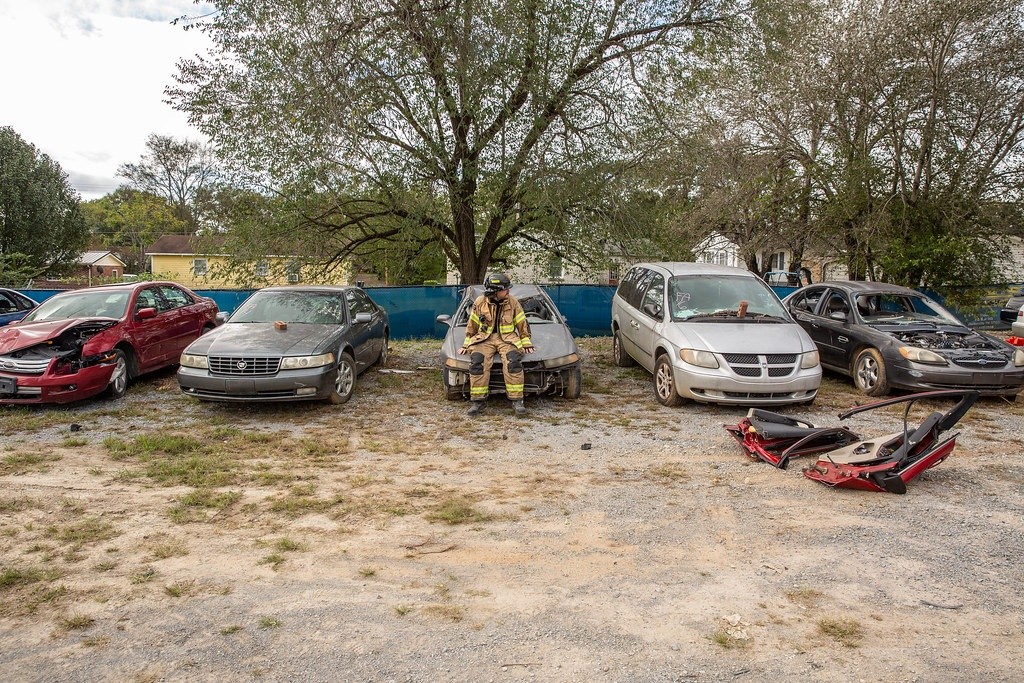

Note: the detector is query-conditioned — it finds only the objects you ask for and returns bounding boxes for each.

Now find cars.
[610,262,822,408]
[771,282,1024,401]
[0,281,225,405]
[180,284,392,407]
[0,288,40,327]
[436,283,581,401]
[1011,306,1024,338]
[1000,295,1024,325]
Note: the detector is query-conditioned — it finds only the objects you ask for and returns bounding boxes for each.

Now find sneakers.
[467,400,487,415]
[511,397,526,415]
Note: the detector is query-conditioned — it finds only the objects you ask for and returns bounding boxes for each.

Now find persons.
[457,273,536,415]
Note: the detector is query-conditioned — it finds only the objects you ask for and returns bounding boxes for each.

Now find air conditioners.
[288,273,298,282]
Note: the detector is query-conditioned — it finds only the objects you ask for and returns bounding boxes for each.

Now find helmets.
[484,273,513,296]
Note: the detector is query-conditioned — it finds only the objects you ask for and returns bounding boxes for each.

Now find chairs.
[0,300,11,312]
[828,297,848,314]
[136,296,149,313]
